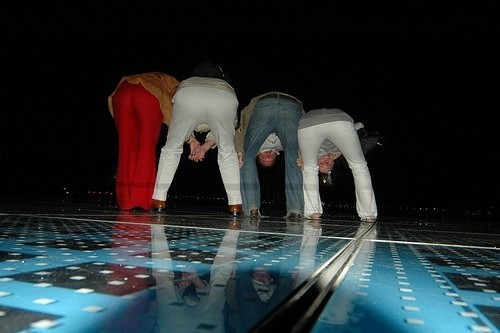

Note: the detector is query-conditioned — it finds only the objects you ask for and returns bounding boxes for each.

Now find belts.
[258,94,302,105]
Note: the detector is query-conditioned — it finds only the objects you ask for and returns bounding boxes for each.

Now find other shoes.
[153,199,166,213]
[229,205,242,215]
[250,209,261,220]
[286,224,302,232]
[132,207,152,214]
[307,220,321,229]
[362,218,376,222]
[227,220,242,230]
[289,213,302,222]
[151,216,166,224]
[306,213,320,219]
[249,221,258,227]
[361,223,376,226]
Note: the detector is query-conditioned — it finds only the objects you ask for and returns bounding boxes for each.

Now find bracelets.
[186,136,190,142]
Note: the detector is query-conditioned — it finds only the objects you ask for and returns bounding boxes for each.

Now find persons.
[112,208,378,333]
[109,72,180,212]
[297,108,378,222]
[234,92,303,220]
[152,62,244,217]
